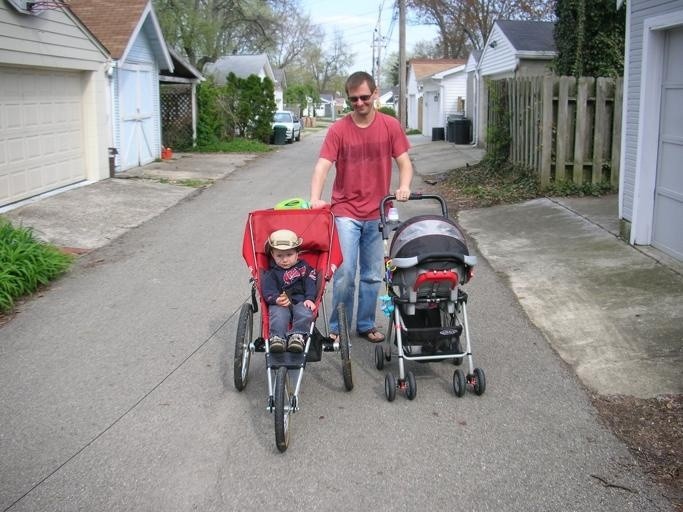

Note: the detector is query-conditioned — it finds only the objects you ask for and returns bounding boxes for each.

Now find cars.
[263,110,303,142]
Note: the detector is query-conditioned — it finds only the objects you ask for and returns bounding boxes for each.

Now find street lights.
[330,100,338,120]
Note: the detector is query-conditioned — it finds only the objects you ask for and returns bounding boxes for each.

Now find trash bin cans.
[273,125,287,144]
[446,114,471,144]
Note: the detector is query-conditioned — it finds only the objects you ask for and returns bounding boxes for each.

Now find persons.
[258,230,316,353]
[309,71,414,344]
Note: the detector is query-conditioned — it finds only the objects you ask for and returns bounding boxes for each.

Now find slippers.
[359,327,385,343]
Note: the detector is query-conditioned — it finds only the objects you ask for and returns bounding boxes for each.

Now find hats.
[264,229,303,256]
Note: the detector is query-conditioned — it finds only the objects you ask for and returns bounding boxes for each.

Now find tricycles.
[231,198,353,454]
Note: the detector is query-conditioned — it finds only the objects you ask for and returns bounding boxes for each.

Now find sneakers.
[287,334,305,352]
[270,336,286,352]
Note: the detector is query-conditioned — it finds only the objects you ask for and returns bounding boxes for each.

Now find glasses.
[348,92,373,102]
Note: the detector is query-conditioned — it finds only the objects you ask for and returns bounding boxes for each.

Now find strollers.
[370,192,491,402]
